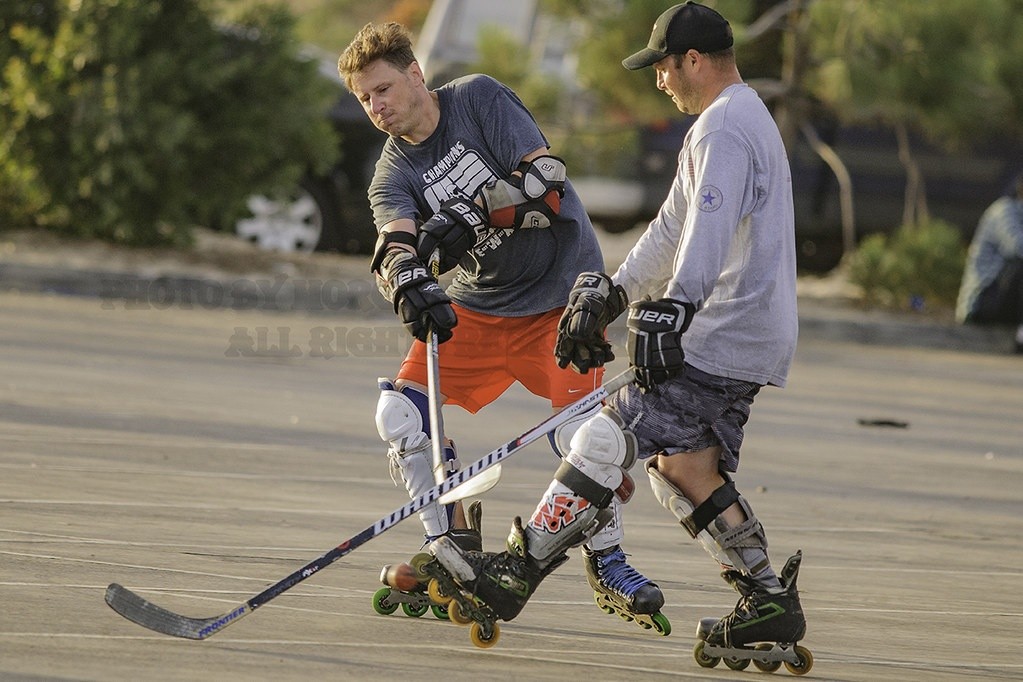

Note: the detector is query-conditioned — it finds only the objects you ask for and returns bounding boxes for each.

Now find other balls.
[388,564,416,594]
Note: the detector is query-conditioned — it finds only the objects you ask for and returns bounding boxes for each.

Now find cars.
[225,48,380,255]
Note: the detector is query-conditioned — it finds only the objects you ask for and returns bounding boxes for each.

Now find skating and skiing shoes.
[420,514,571,648]
[579,547,671,636]
[372,500,485,619]
[694,551,814,676]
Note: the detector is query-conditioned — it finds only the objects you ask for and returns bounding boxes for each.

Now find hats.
[622,0,733,71]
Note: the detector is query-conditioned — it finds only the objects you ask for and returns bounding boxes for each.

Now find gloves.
[624,293,696,394]
[554,271,628,375]
[385,261,458,344]
[418,197,491,276]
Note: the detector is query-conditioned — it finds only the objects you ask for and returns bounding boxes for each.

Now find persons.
[336,21,671,637]
[951,177,1022,356]
[421,0,814,675]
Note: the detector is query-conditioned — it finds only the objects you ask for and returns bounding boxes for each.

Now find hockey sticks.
[104,363,641,640]
[426,239,502,504]
